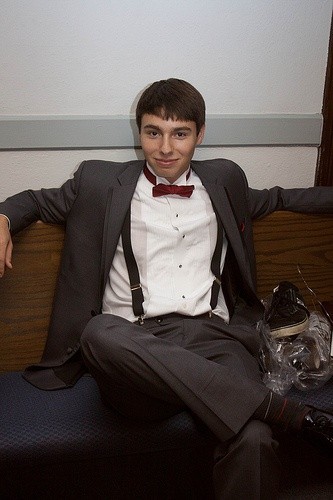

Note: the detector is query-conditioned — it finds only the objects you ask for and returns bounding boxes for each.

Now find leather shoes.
[298,404,333,454]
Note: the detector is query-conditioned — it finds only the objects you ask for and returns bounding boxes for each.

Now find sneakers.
[266,280,309,337]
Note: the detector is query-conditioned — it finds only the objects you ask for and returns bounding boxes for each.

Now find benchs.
[0,211,333,500]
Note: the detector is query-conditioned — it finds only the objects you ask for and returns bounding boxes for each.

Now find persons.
[1,77,333,500]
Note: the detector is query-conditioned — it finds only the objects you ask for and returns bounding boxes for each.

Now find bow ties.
[143,163,195,198]
